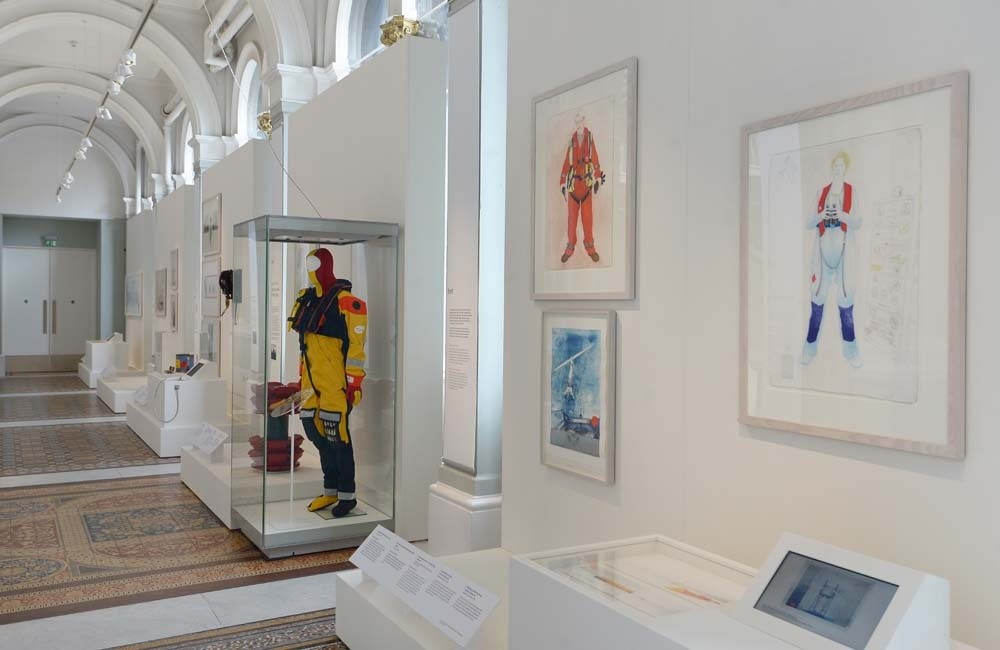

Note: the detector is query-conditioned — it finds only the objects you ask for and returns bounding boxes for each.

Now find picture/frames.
[540,309,617,484]
[739,69,970,459]
[124,273,144,317]
[199,192,222,378]
[155,249,179,333]
[531,56,638,300]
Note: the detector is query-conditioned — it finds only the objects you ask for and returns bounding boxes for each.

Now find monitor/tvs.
[186,362,203,377]
[107,334,114,341]
[753,551,900,650]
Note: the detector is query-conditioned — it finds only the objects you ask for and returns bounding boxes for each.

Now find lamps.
[95,106,113,121]
[106,49,136,95]
[55,172,74,202]
[75,138,93,160]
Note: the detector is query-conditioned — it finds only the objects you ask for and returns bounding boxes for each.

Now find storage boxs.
[232,216,398,559]
